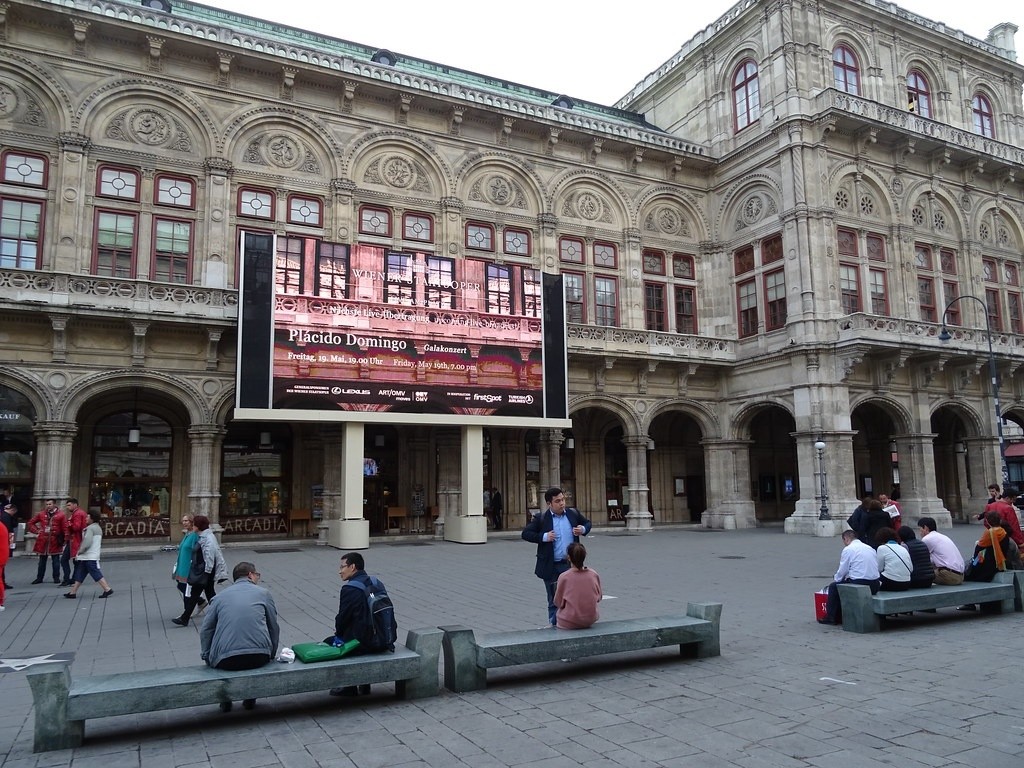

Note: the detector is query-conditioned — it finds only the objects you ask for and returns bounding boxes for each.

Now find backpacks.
[343,576,397,648]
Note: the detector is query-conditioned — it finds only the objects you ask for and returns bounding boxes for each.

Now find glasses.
[340,565,352,570]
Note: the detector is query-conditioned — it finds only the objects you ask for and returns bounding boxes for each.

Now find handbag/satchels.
[814,585,829,619]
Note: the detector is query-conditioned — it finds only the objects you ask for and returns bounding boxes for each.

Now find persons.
[521,488,593,623]
[0,486,114,612]
[819,483,1024,625]
[200,562,280,712]
[171,516,228,626]
[176,512,209,619]
[322,552,388,697]
[490,487,503,530]
[553,542,603,630]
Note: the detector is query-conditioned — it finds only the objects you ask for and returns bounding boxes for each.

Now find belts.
[945,566,964,576]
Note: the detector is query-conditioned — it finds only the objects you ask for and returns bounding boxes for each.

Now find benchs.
[439,601,724,694]
[26,627,445,753]
[836,572,1015,633]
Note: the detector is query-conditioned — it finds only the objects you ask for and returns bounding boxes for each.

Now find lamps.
[374,422,384,448]
[647,429,655,451]
[259,423,271,445]
[565,429,575,449]
[128,388,141,447]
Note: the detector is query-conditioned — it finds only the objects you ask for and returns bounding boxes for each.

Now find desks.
[286,508,312,537]
[385,506,408,534]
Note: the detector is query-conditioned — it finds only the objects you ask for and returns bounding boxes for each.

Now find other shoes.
[220,702,231,713]
[64,591,76,598]
[0,606,5,611]
[31,579,43,584]
[60,580,76,586]
[172,617,188,626]
[99,588,113,598]
[330,685,358,698]
[818,615,836,625]
[243,699,254,709]
[359,684,370,695]
[4,583,13,589]
[956,604,977,610]
[54,579,60,583]
[195,601,208,614]
[916,608,936,614]
